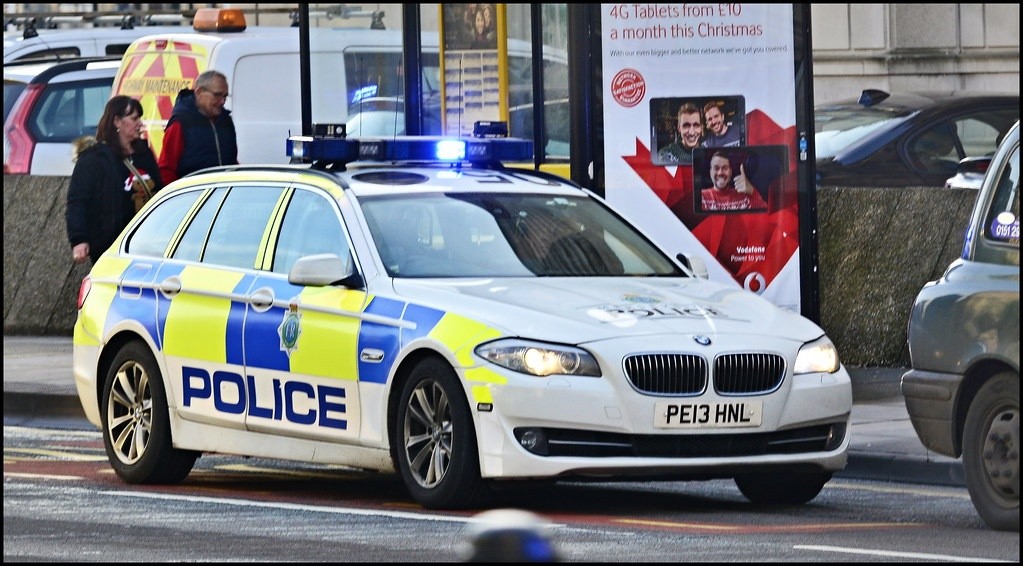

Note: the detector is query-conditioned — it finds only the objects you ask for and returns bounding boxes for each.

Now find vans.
[110,8,570,181]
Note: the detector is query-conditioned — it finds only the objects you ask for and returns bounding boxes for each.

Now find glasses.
[203,87,229,99]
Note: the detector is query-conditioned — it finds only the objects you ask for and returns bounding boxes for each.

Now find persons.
[63,96,166,268]
[701,101,740,147]
[700,150,768,211]
[658,103,706,164]
[460,4,498,50]
[154,69,236,186]
[509,204,555,262]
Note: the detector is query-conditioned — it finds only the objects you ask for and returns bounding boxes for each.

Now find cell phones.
[650,96,790,213]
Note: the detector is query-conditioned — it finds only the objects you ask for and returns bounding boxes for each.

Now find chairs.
[53,99,87,137]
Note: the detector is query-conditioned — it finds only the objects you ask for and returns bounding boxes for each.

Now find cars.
[900,119,1020,531]
[815,89,1020,187]
[73,136,852,510]
[4,24,197,175]
